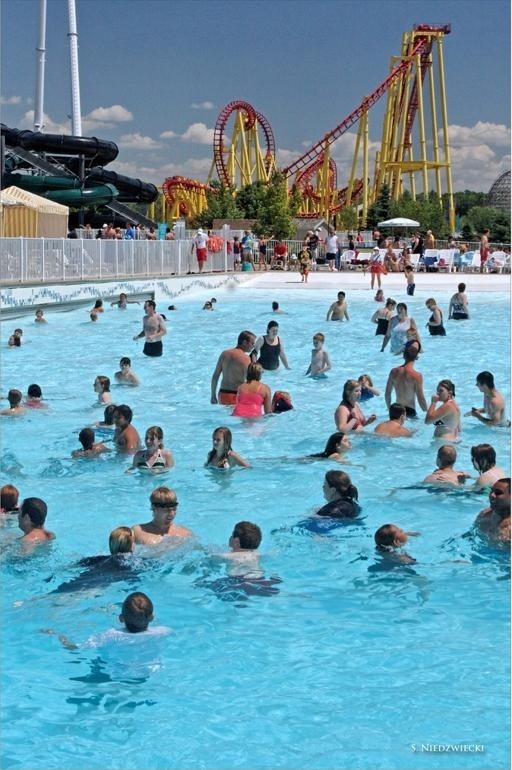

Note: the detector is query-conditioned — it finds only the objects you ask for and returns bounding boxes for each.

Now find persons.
[1,220,509,689]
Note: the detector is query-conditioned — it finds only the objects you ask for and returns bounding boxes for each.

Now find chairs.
[268,242,510,273]
